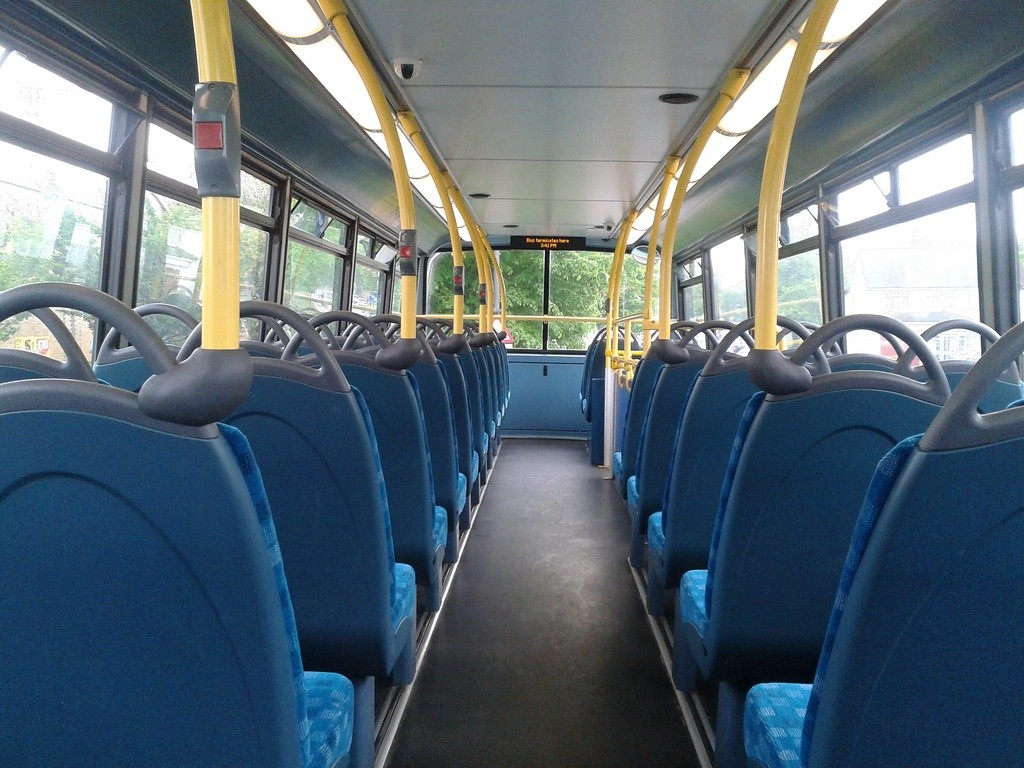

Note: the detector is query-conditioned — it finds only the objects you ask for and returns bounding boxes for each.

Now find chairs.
[578,319,1023,768]
[0,282,508,768]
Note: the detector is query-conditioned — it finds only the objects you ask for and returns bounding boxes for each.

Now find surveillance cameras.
[392,59,420,80]
